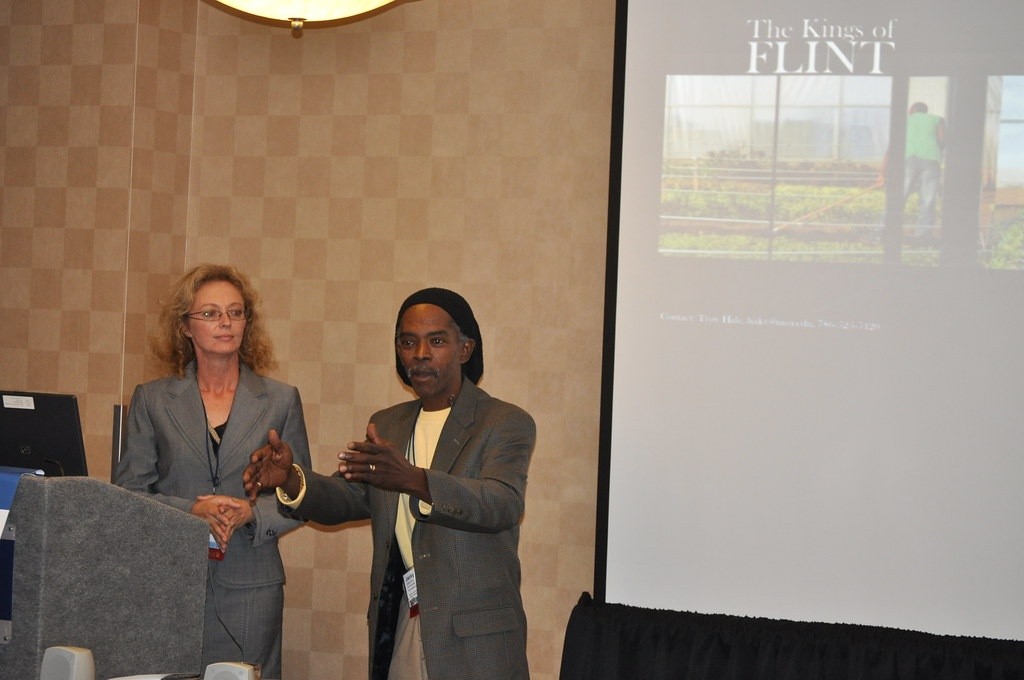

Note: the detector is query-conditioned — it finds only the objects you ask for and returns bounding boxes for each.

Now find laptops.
[0,390,90,476]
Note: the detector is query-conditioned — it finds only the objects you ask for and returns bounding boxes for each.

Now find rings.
[369,463,375,473]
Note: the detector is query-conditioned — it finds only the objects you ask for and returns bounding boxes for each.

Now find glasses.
[183,309,249,321]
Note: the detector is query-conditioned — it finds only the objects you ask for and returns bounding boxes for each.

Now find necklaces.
[207,416,220,444]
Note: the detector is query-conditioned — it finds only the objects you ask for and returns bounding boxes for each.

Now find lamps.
[200,0,418,32]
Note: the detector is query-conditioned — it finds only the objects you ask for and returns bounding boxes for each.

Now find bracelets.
[284,468,303,502]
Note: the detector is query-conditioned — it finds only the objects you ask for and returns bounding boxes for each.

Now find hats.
[394,288,484,386]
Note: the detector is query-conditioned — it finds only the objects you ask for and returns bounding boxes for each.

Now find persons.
[879,100,948,242]
[114,265,312,680]
[243,286,537,680]
